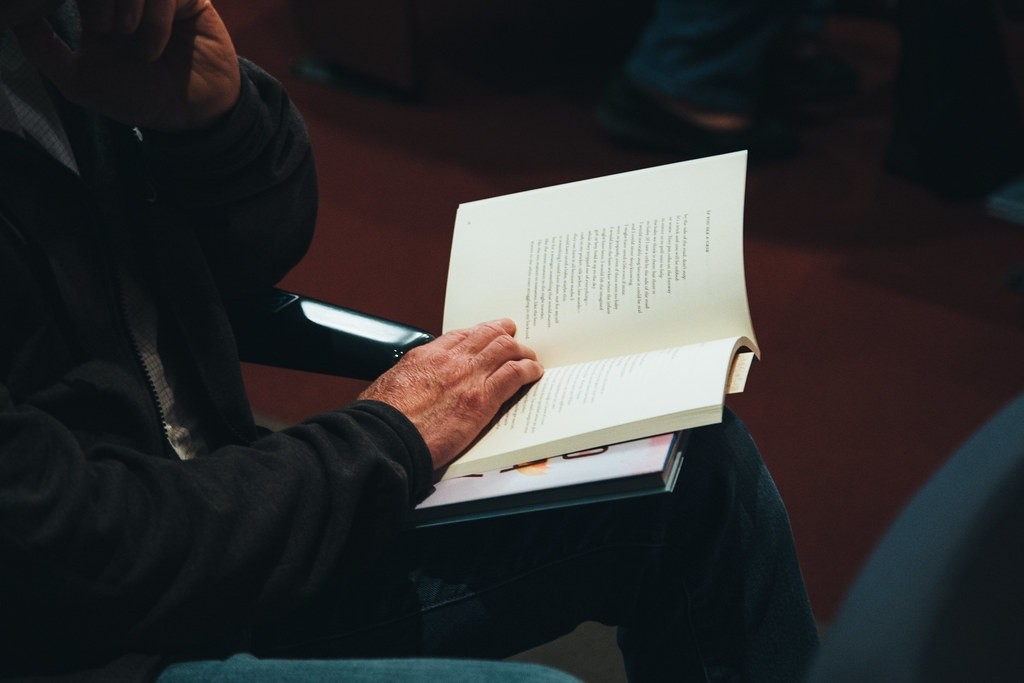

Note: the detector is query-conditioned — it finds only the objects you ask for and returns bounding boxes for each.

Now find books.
[411,150,761,529]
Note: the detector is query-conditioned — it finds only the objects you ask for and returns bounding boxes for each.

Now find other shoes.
[595,53,858,166]
[984,173,1023,224]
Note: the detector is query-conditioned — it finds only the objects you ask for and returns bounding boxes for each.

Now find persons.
[0,0,819,683]
